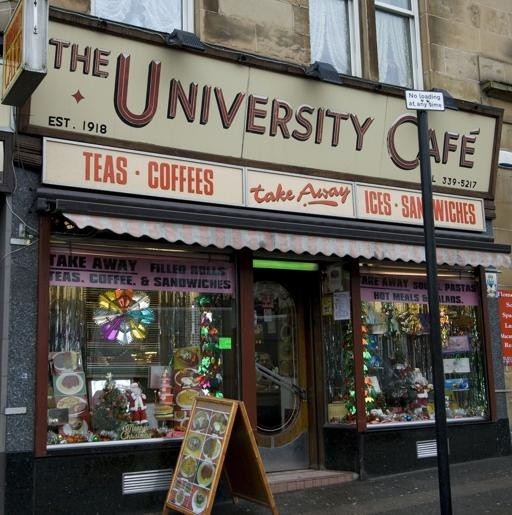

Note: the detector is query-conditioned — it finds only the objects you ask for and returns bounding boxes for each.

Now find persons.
[158,369,174,406]
[255,352,280,389]
[129,383,149,425]
